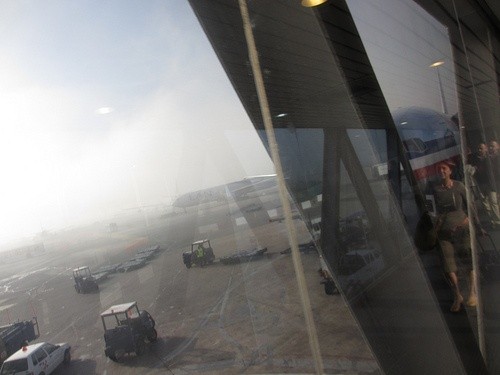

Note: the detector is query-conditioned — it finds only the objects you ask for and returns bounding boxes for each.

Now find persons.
[414,138,500,313]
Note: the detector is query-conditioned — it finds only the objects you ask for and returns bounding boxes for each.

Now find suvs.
[0,342,73,375]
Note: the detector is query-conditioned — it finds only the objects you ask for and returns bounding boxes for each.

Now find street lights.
[430,56,452,119]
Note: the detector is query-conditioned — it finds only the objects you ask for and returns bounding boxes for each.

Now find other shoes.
[468,292,481,306]
[452,294,463,312]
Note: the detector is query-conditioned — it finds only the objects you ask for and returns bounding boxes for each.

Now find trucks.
[0,315,40,365]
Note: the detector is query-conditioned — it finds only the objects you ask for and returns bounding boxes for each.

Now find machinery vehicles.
[72,266,99,295]
[102,299,158,363]
[182,238,215,270]
